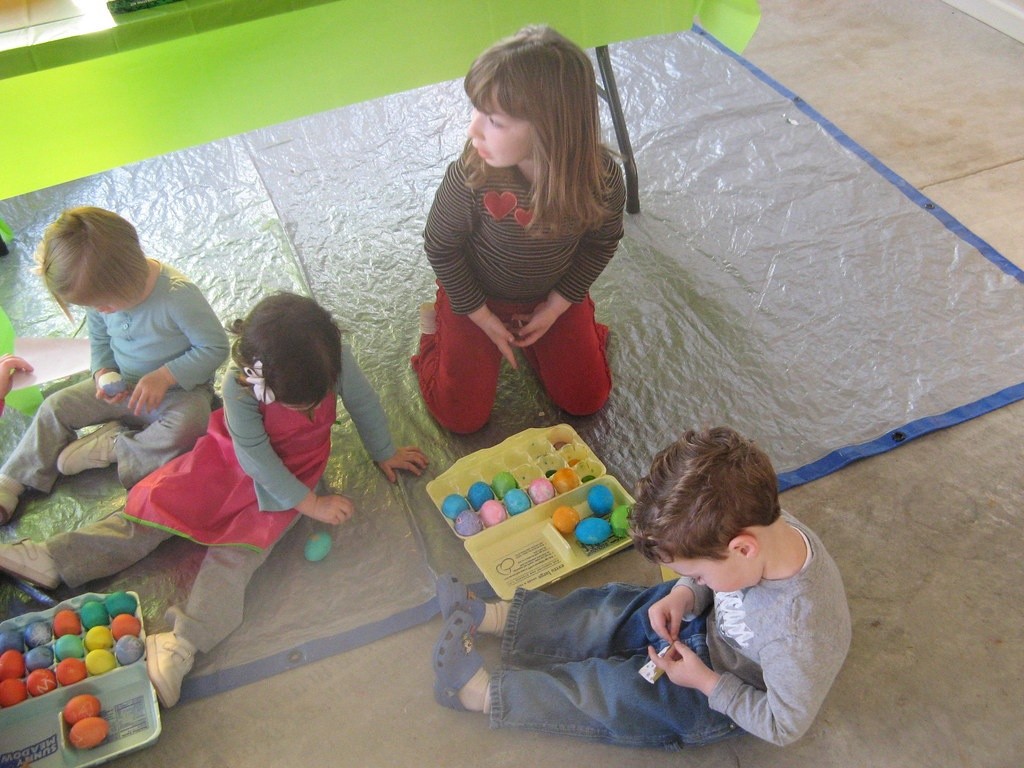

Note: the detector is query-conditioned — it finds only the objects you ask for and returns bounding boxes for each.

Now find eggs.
[303,529,332,561]
[441,471,633,544]
[98,372,128,397]
[0,591,143,749]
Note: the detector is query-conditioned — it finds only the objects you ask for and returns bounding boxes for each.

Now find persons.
[409,23,626,435]
[1,205,430,709]
[433,427,852,752]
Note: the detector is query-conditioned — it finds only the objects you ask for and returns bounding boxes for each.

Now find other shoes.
[420,302,438,335]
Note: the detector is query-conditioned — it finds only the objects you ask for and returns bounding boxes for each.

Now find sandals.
[433,610,485,712]
[436,572,487,635]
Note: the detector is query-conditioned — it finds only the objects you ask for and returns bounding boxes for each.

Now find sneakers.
[145,632,195,708]
[0,485,19,526]
[57,420,129,475]
[0,538,62,591]
